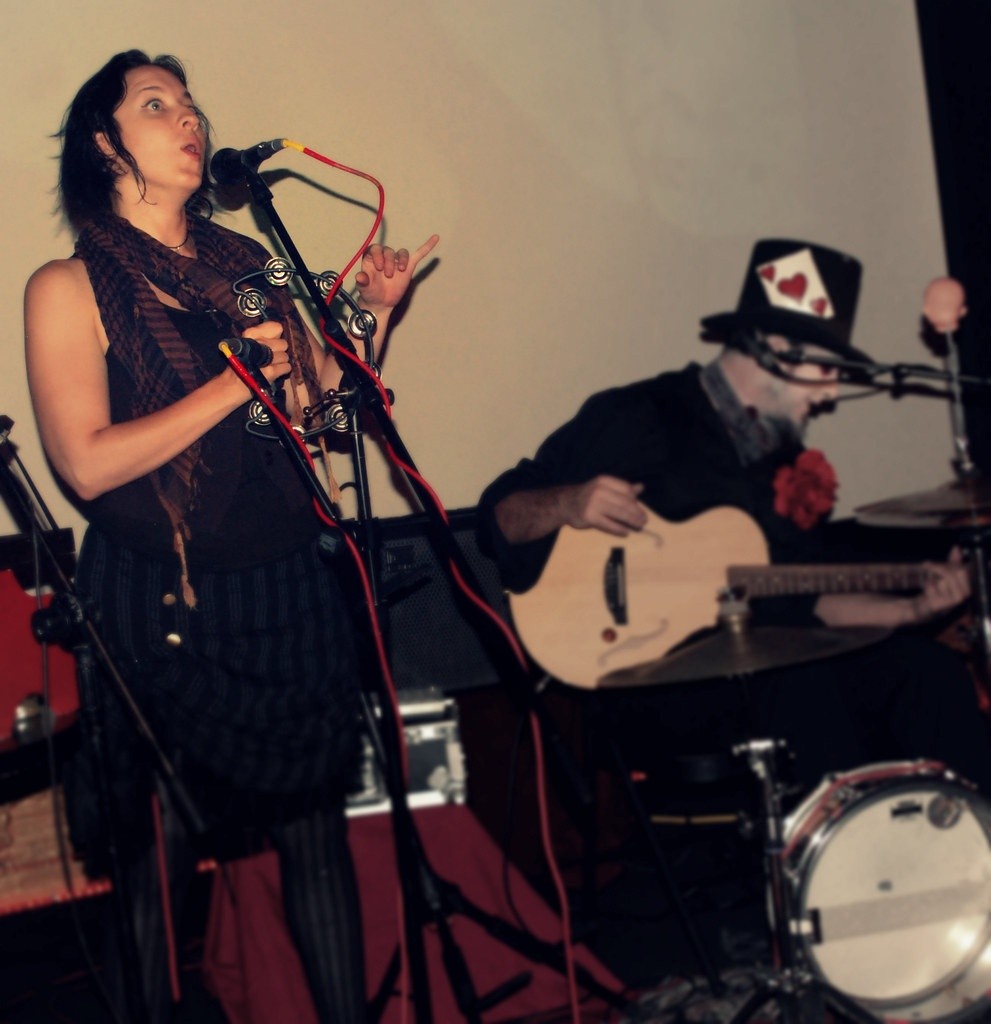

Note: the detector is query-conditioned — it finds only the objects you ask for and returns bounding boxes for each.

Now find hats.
[701,237,878,381]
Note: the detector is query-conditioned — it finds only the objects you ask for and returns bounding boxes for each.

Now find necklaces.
[167,233,189,251]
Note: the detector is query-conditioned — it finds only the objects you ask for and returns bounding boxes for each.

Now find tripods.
[641,737,865,1021]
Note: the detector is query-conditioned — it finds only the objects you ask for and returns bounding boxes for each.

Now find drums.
[766,758,991,1024]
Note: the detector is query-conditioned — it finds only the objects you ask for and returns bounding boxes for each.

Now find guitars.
[507,492,979,691]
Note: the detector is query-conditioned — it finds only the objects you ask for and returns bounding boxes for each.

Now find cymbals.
[852,472,991,520]
[232,256,379,449]
[594,611,894,688]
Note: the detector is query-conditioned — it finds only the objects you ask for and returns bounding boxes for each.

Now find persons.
[22,48,440,1024]
[476,239,974,1022]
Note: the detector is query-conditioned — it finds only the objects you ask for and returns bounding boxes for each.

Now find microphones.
[221,337,274,368]
[211,139,290,186]
[705,313,781,371]
